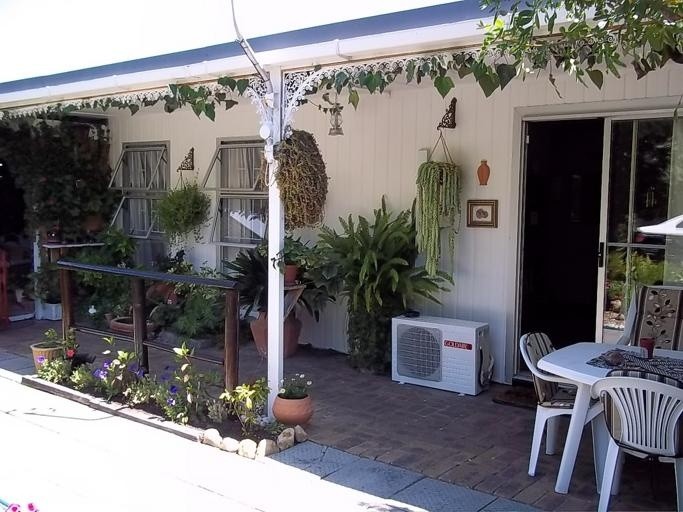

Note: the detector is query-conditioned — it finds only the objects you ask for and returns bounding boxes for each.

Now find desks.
[536,338,683,388]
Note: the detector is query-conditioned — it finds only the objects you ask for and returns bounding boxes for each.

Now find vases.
[272,392,318,431]
[477,160,490,186]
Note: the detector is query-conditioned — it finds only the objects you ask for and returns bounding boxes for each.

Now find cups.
[639,338,655,359]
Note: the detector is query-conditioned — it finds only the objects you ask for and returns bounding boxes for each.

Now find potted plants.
[416,160,464,279]
[319,202,416,371]
[33,263,64,320]
[31,327,63,374]
[261,237,318,286]
[221,238,300,358]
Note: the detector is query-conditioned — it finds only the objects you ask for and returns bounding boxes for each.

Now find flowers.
[280,372,312,397]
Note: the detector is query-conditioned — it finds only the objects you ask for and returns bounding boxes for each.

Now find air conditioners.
[390,314,494,394]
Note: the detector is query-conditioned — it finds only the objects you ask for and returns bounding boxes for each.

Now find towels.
[586,347,683,382]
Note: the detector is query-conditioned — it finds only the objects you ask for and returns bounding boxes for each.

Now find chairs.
[589,376,683,507]
[616,283,683,352]
[518,325,623,498]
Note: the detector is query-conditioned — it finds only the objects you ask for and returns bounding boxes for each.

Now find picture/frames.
[466,200,497,227]
